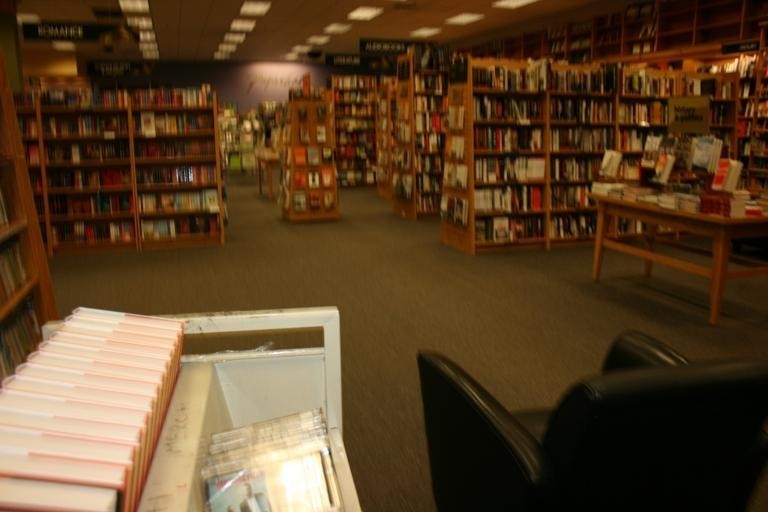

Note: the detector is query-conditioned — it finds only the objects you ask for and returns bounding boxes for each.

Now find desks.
[256,154,280,201]
[587,190,767,326]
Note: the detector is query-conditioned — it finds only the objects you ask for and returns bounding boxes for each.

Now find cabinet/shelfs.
[0,85,229,383]
[282,0,767,256]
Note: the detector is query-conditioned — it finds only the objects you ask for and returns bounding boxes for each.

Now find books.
[0,306,344,511]
[277,88,334,211]
[334,77,387,186]
[0,198,41,378]
[220,101,280,171]
[618,54,767,233]
[11,83,220,243]
[392,47,469,228]
[471,62,617,241]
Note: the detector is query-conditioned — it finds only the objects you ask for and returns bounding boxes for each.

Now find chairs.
[418,329,768,512]
